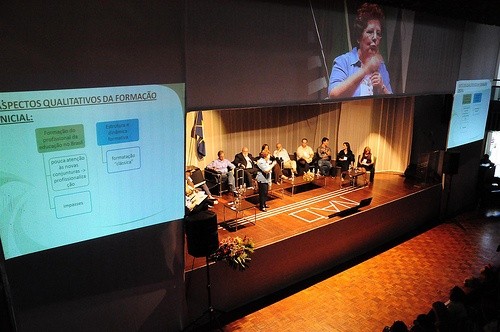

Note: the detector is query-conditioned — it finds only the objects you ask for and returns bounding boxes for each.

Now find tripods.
[181,255,243,332]
[434,175,466,232]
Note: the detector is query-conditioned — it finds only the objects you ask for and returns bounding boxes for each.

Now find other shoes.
[262,209,267,212]
[228,186,233,192]
[266,206,269,208]
[234,192,238,197]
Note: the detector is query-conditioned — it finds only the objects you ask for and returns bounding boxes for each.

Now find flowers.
[218,235,255,273]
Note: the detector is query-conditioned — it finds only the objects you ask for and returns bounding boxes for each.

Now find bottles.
[317,169,319,179]
[291,173,295,184]
[238,195,241,205]
[243,183,246,192]
[235,199,239,208]
[349,165,351,173]
[240,185,242,194]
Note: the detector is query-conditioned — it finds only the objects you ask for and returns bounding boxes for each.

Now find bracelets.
[381,84,386,91]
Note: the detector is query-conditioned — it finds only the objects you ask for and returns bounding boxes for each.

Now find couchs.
[203,166,242,196]
[232,161,269,187]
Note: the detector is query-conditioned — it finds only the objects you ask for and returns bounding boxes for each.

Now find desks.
[240,175,325,200]
[223,198,256,232]
[340,171,364,190]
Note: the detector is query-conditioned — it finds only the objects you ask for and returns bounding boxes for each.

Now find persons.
[184,138,375,212]
[383,264,500,332]
[328,3,394,99]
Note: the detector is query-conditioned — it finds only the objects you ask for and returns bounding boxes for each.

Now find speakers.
[184,210,219,258]
[446,153,460,175]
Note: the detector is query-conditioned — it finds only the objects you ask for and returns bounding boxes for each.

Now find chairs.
[257,152,377,183]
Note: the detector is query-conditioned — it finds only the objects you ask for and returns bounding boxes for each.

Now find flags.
[191,110,205,159]
